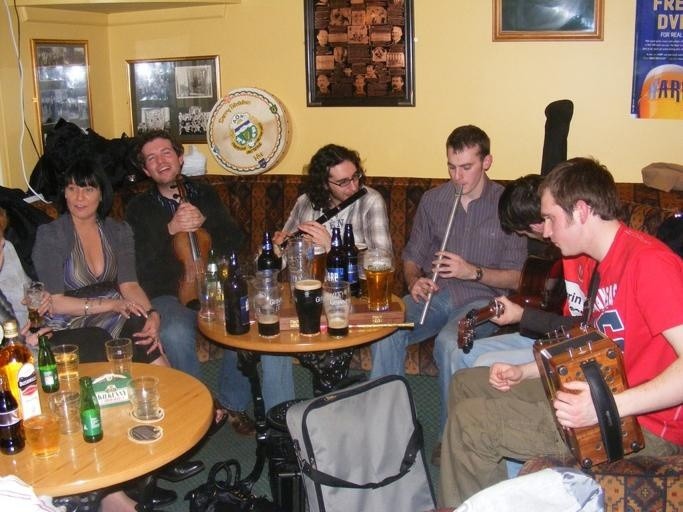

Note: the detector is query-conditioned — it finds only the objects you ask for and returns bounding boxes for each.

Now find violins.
[170,175,215,310]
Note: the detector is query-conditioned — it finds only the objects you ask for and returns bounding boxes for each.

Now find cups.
[251,279,283,340]
[104,336,135,382]
[291,278,322,337]
[286,235,315,278]
[50,343,81,382]
[22,279,47,332]
[46,389,82,436]
[356,250,394,313]
[124,374,161,419]
[324,279,352,340]
[194,272,219,321]
[23,411,62,459]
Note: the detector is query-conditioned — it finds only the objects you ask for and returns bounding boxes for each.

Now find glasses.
[326,166,364,189]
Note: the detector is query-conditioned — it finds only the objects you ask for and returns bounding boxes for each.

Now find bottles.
[1,380,27,455]
[78,377,104,445]
[223,250,249,335]
[342,223,358,295]
[325,226,345,286]
[256,229,285,273]
[37,335,59,393]
[1,320,42,419]
[206,246,217,276]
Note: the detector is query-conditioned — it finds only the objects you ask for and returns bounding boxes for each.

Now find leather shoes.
[226,408,259,437]
[128,483,178,507]
[155,458,205,483]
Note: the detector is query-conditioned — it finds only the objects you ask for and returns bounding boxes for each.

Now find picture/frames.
[31,36,94,157]
[491,0,605,42]
[124,53,222,144]
[303,1,415,107]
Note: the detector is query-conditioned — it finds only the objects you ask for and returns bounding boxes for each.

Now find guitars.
[457,255,565,353]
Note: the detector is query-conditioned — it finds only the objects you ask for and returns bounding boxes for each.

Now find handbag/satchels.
[283,370,439,512]
[184,457,257,512]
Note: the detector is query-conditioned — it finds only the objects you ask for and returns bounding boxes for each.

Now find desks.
[197,280,406,511]
[0,360,214,512]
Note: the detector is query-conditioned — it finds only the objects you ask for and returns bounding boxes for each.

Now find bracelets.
[475,266,483,282]
[147,308,156,314]
[84,298,89,316]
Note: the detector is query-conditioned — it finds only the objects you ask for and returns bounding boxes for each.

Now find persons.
[473,174,573,479]
[31,161,172,368]
[1,206,55,362]
[125,130,257,437]
[314,3,409,99]
[260,143,408,417]
[436,157,683,511]
[369,124,530,467]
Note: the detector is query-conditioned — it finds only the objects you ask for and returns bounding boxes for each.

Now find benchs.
[0,174,682,510]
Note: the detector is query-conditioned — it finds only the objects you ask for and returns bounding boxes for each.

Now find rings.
[126,304,132,308]
[48,298,52,303]
[148,335,156,342]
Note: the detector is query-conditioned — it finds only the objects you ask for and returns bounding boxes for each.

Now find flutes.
[280,188,367,249]
